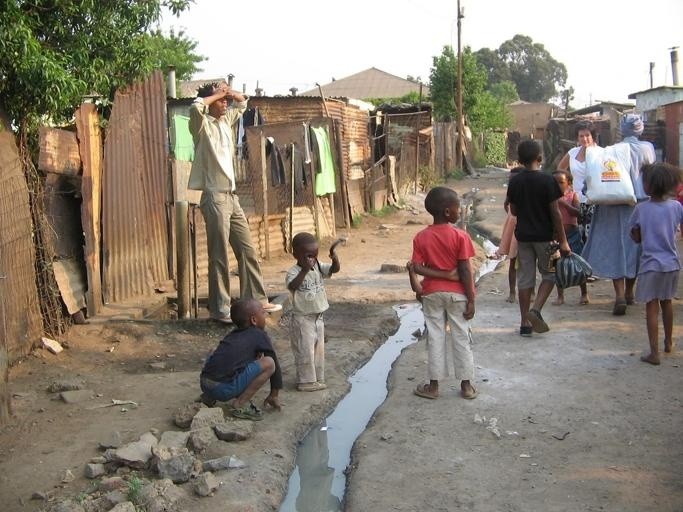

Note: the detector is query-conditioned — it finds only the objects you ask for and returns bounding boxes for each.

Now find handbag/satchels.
[585,141,638,207]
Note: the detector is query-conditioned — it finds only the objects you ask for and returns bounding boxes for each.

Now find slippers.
[463,383,479,400]
[413,383,441,399]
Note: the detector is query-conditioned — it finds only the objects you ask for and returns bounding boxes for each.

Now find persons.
[499,112,682,364]
[195,297,283,420]
[189,82,283,323]
[286,233,345,392]
[408,188,479,400]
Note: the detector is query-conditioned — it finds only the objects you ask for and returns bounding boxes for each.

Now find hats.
[618,112,645,138]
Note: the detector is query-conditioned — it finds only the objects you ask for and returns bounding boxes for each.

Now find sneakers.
[520,326,533,337]
[217,313,235,325]
[297,380,326,392]
[613,293,635,316]
[229,399,265,421]
[260,302,283,312]
[526,309,551,335]
[194,392,216,407]
